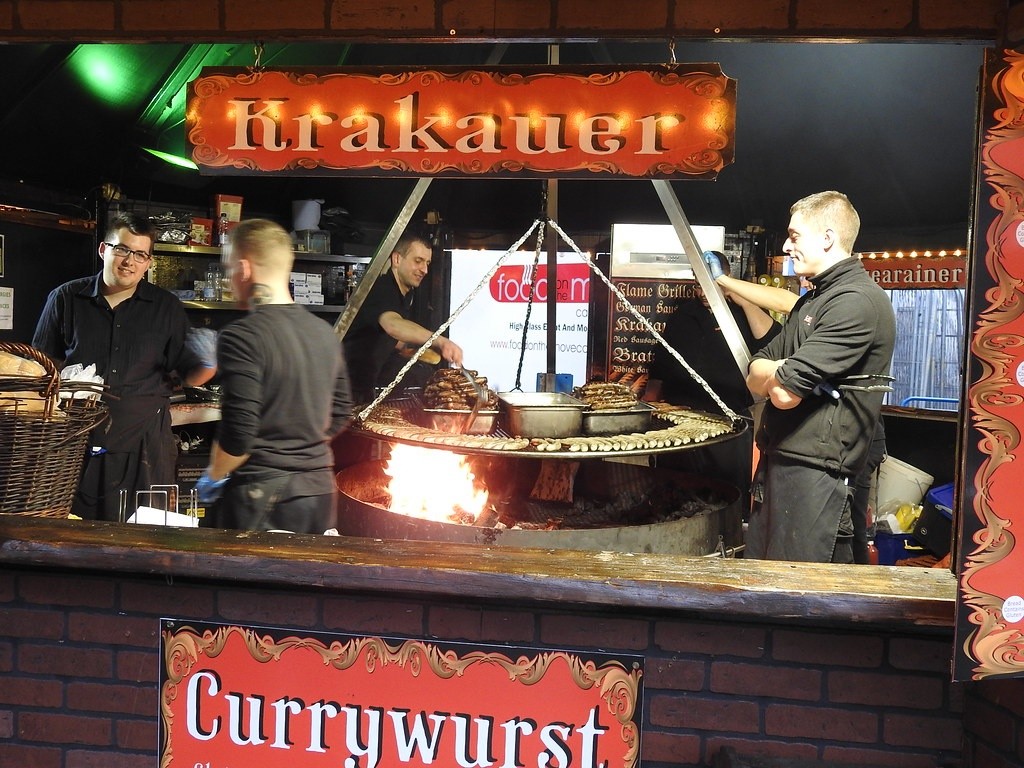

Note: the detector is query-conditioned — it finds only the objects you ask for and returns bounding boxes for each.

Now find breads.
[0,350,63,411]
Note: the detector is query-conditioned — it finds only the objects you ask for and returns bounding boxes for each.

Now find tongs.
[456,359,489,404]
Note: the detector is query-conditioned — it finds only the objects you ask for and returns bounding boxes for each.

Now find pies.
[408,347,441,364]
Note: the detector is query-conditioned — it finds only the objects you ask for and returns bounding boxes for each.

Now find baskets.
[0,343,120,517]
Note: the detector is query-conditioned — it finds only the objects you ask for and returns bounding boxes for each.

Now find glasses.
[104,243,150,263]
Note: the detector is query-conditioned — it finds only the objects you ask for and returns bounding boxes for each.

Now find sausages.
[350,368,735,452]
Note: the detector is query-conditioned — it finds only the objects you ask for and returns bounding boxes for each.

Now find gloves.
[195,476,229,504]
[185,327,217,369]
[704,250,723,280]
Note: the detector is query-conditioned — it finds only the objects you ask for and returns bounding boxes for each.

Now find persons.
[32,210,219,523]
[703,251,885,564]
[193,223,354,534]
[642,250,770,524]
[341,227,464,405]
[746,189,895,564]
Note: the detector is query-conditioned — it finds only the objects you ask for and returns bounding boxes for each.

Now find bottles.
[758,274,785,287]
[204,266,215,302]
[214,267,223,302]
[217,213,229,247]
[335,272,345,304]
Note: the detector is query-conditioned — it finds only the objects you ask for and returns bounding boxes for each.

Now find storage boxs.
[910,482,955,561]
[288,272,324,305]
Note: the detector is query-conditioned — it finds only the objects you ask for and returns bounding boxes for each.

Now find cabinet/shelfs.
[150,242,374,427]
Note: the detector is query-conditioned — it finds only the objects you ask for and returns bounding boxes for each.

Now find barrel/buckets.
[868,453,934,517]
